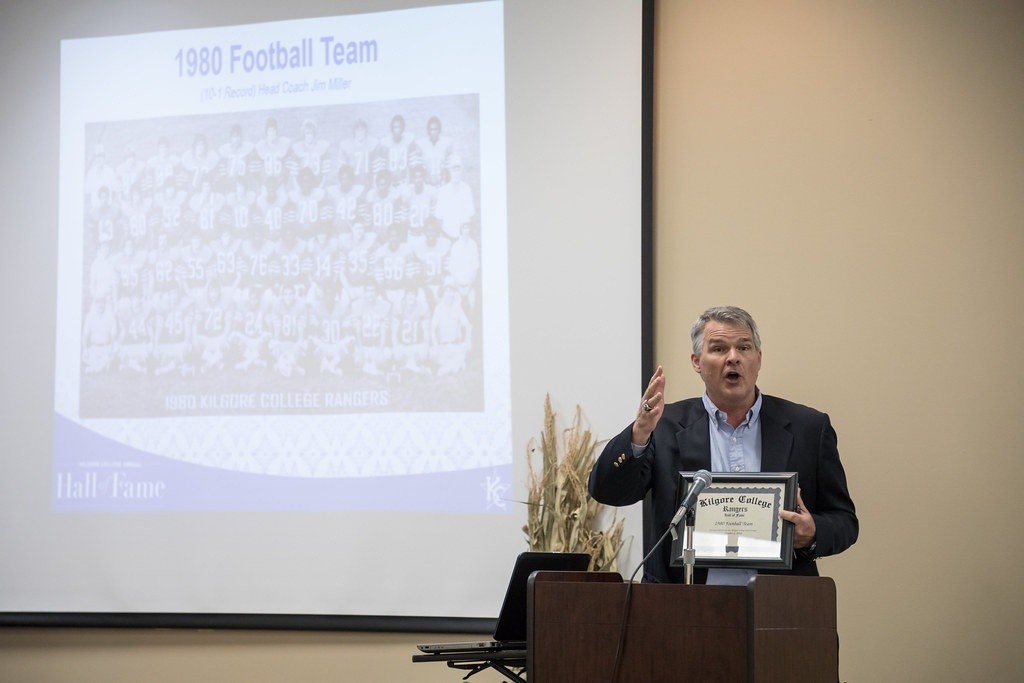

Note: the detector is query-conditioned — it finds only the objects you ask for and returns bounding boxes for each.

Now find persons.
[587,305,859,587]
[85,115,478,392]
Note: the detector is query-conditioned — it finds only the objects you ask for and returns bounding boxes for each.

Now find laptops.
[416,551,592,653]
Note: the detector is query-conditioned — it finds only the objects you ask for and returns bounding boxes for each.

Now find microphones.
[671,470,713,527]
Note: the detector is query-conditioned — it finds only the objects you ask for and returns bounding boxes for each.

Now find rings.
[642,400,653,412]
